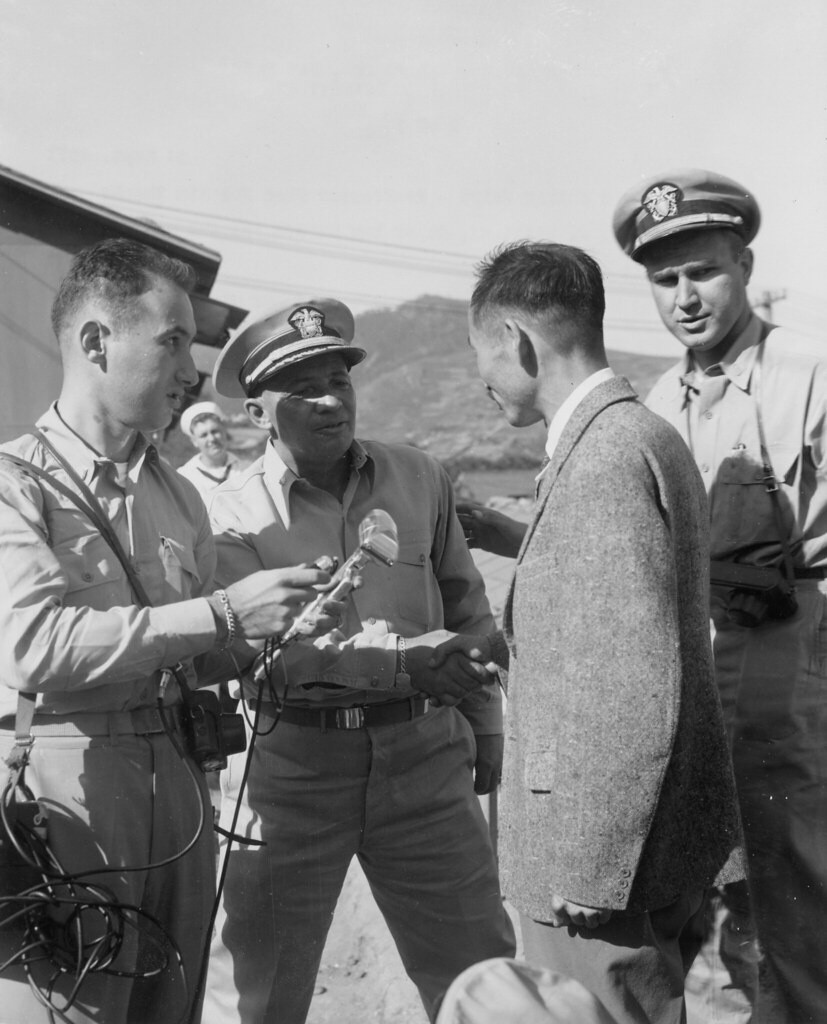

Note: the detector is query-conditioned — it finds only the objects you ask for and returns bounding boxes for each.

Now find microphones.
[253,507,400,689]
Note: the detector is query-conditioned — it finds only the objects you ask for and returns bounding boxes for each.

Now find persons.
[426,236,745,1024]
[0,237,332,1024]
[209,297,516,1024]
[609,170,827,1024]
[174,399,255,537]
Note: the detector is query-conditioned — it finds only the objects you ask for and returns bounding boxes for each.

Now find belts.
[0,705,183,737]
[246,694,433,729]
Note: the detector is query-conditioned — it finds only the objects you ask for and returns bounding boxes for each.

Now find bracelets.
[396,634,413,695]
[212,590,237,653]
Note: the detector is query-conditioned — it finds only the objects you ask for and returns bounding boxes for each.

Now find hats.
[211,291,373,396]
[180,400,225,433]
[610,167,766,257]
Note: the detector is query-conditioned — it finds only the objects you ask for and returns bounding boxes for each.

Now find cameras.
[707,560,799,629]
[183,690,247,772]
